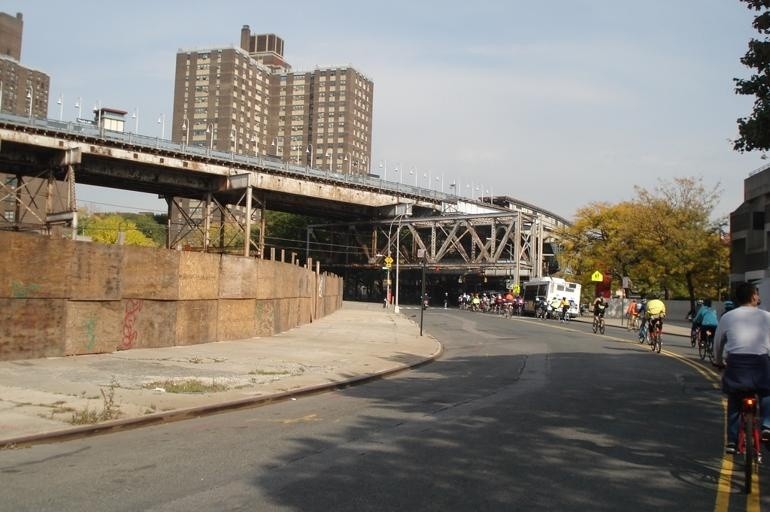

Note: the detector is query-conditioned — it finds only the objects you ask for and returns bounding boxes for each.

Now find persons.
[558,297,570,323]
[537,296,549,319]
[457,291,524,318]
[550,297,558,317]
[627,294,665,342]
[593,291,608,324]
[686,298,734,353]
[714,281,770,454]
[423,293,429,304]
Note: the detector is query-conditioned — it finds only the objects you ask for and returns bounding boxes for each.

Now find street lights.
[384,211,413,313]
[395,222,416,313]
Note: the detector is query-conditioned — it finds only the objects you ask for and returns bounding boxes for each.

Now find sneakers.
[726,444,736,454]
[761,427,770,442]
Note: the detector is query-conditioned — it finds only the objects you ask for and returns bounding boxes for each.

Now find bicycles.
[695,324,717,366]
[627,312,661,353]
[711,362,770,493]
[460,300,569,323]
[590,310,605,334]
[688,317,704,348]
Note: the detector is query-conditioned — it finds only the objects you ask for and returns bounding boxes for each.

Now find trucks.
[522,276,582,317]
